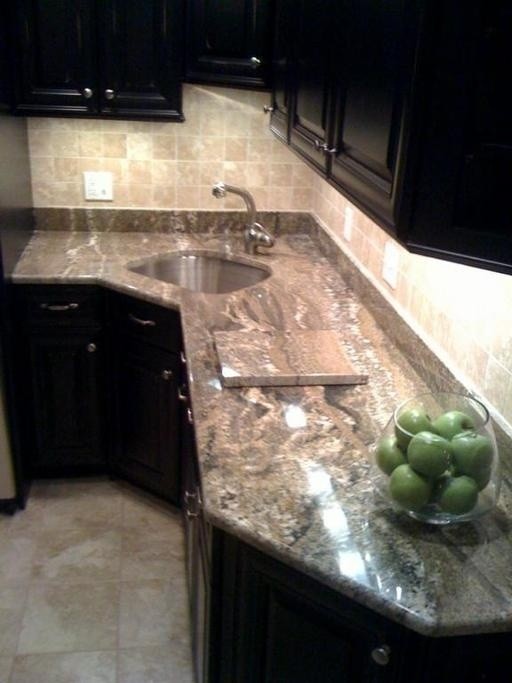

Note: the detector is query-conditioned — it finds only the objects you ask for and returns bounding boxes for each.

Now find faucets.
[211,182,277,254]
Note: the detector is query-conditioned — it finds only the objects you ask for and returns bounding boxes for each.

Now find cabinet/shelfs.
[1,228,402,501]
[181,363,512,683]
[1,0,277,124]
[266,1,511,275]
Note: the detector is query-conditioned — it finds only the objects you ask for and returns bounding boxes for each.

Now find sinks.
[124,248,275,296]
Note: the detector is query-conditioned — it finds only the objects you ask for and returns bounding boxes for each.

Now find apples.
[376,407,496,516]
